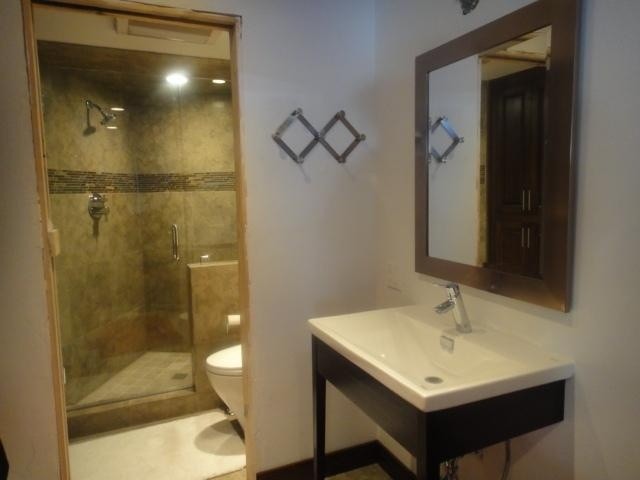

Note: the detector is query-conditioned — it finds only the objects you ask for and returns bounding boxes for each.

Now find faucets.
[434,285,471,333]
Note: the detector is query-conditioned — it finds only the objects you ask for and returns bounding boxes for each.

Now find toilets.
[204,344,244,431]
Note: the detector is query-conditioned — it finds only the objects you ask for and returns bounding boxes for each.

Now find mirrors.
[34,39,240,415]
[413,0,585,315]
[23,0,263,480]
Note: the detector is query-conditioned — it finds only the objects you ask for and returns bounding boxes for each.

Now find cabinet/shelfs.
[488,65,548,279]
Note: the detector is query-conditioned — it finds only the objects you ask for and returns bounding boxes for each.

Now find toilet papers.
[224,314,241,337]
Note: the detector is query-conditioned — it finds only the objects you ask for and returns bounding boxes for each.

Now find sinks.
[308,306,575,413]
